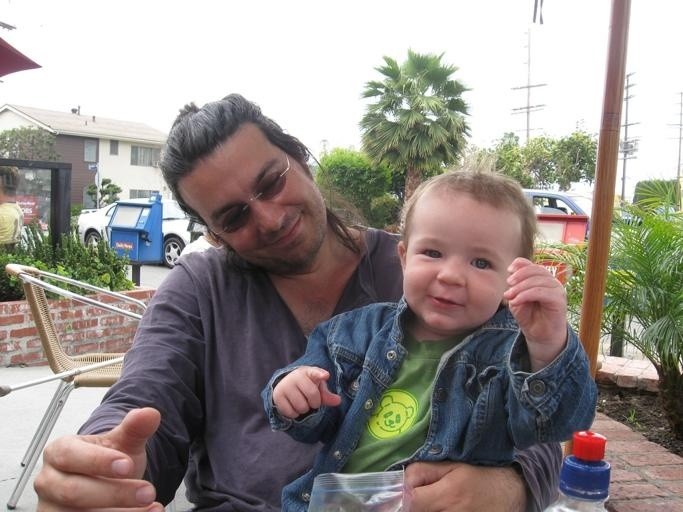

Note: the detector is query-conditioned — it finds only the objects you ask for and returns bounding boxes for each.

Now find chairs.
[0,262,153,512]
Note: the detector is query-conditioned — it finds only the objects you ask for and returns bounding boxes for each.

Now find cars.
[76,197,210,269]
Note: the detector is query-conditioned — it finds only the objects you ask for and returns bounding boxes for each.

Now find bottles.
[542,430,612,512]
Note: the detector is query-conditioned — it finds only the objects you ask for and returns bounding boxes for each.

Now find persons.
[0,165,24,244]
[29,93,564,512]
[258,169,596,512]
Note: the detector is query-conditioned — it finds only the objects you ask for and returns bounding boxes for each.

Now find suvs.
[521,186,675,252]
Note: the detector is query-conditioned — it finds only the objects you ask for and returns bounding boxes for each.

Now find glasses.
[207,153,292,238]
